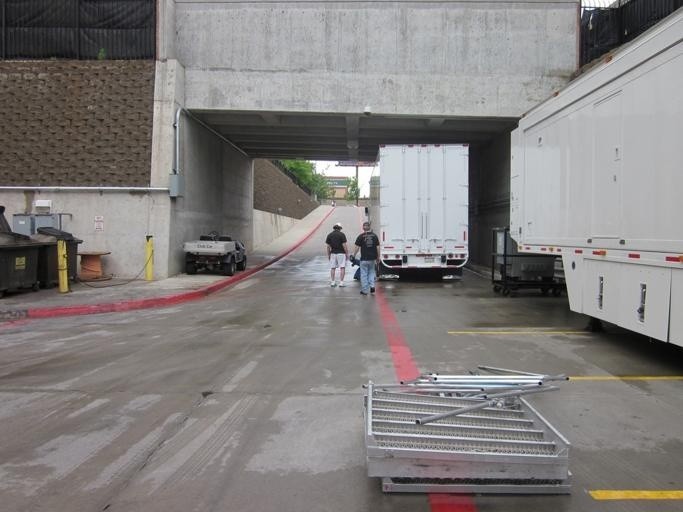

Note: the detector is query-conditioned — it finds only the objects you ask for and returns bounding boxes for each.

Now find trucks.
[371,143,472,281]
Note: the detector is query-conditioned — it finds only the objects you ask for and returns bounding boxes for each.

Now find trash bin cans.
[0,227,83,299]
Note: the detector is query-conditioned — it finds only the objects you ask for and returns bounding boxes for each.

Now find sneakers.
[339,283,346,287]
[331,281,337,287]
[370,288,375,295]
[360,291,367,296]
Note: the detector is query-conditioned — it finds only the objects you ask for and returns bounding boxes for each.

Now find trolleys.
[490,228,567,298]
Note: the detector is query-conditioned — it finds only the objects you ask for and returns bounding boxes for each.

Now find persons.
[352,222,381,297]
[324,222,349,288]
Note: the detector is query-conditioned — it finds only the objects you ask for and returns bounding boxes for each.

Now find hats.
[334,223,342,227]
